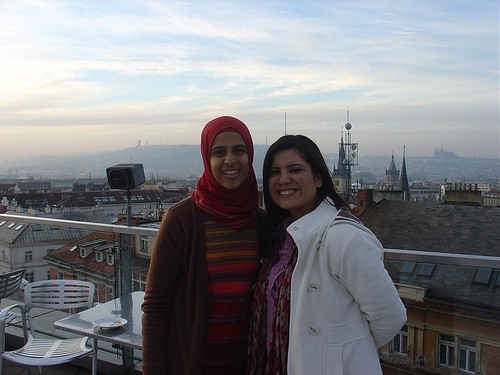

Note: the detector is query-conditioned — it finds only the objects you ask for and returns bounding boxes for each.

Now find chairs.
[0,279,104,375]
[0,268,43,375]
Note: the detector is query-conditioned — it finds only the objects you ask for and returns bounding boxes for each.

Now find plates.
[93,317,127,329]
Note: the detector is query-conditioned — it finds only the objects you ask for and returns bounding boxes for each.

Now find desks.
[53,290,145,375]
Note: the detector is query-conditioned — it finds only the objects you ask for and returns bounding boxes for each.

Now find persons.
[140,116,265,374]
[247,135,408,375]
